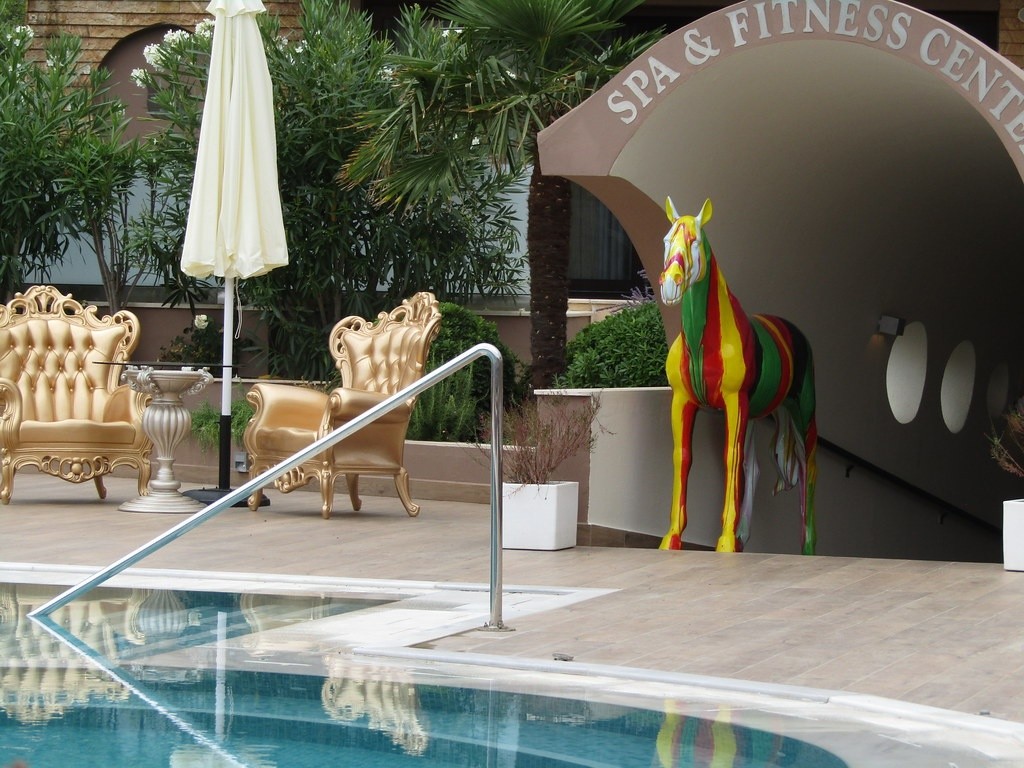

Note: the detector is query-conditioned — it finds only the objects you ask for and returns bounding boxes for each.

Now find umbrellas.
[180,0,289,507]
[169,590,276,768]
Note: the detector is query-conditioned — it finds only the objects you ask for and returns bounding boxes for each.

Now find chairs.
[0,285,152,505]
[242,290,443,520]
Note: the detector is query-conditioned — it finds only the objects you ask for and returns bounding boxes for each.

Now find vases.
[502,481,578,551]
[1002,499,1024,572]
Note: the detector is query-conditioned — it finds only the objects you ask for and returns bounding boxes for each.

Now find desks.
[92,361,245,514]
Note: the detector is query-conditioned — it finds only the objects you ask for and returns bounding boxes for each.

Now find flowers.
[451,387,622,502]
[984,404,1024,478]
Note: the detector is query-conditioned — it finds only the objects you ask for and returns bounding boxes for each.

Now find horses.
[653,193,822,556]
